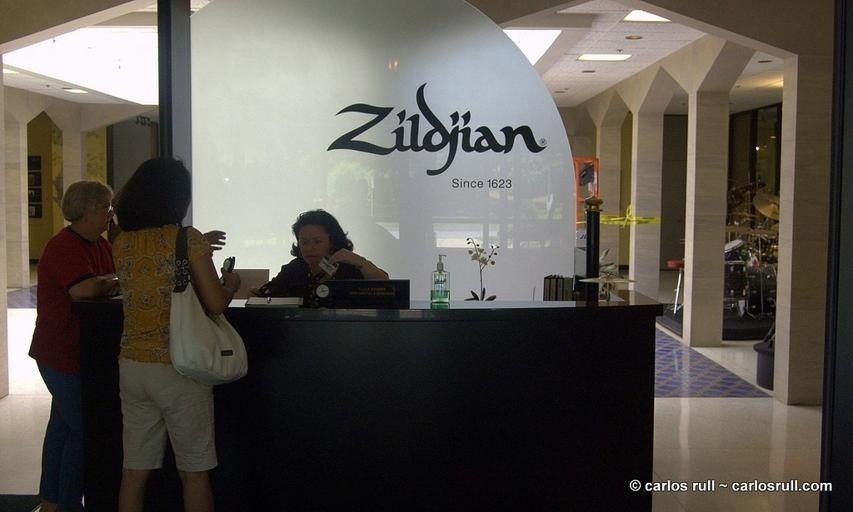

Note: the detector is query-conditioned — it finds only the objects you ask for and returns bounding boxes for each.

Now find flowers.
[464,236,501,303]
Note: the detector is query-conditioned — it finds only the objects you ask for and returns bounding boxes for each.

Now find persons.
[26,182,119,512]
[250,208,392,301]
[109,154,242,511]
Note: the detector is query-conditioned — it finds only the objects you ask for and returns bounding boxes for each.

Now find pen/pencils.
[267,296,271,304]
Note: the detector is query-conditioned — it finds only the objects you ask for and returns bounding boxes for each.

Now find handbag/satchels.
[170,229,249,386]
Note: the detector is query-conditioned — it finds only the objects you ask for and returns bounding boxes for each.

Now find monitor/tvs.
[323,280,409,310]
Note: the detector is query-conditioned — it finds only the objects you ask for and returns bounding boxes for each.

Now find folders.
[550,279,556,300]
[557,279,562,301]
[563,279,573,301]
[544,278,550,301]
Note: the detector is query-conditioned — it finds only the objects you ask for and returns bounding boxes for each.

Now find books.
[244,294,307,308]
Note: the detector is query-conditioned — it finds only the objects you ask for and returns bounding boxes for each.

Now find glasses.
[104,206,114,213]
